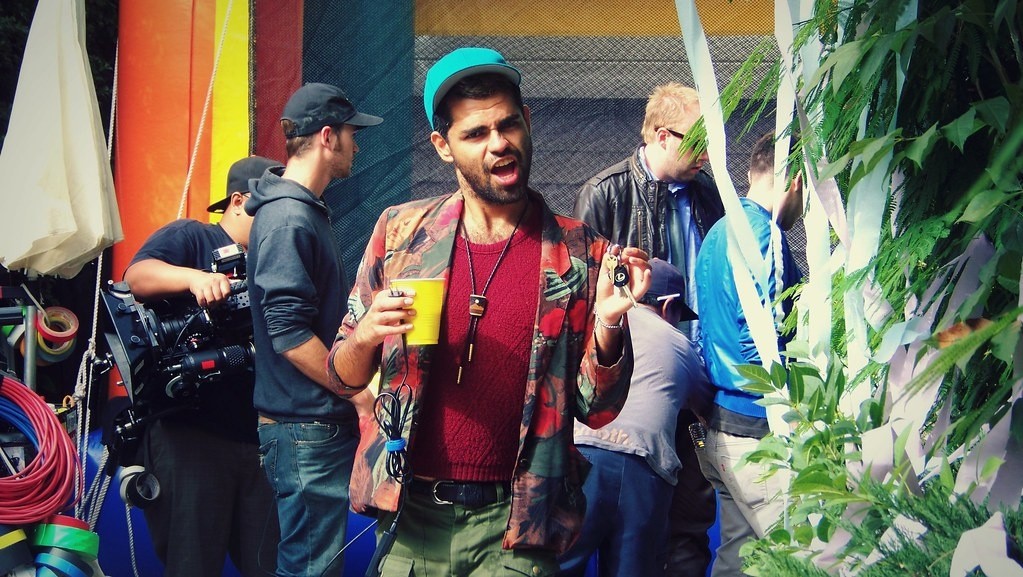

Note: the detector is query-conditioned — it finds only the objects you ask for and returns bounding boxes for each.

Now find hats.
[207,155,287,213]
[424,47,521,131]
[645,257,699,322]
[279,82,384,138]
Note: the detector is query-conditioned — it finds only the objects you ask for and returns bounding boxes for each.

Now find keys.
[607,264,639,309]
[606,253,619,284]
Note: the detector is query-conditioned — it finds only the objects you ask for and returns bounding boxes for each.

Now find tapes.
[119,466,162,509]
[0,307,79,367]
[35,515,100,577]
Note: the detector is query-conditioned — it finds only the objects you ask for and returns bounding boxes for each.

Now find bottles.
[689,422,706,448]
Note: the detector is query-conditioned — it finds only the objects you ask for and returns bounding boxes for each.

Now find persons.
[555,82,727,577]
[688,129,802,577]
[244,82,384,577]
[123,155,283,577]
[327,47,652,577]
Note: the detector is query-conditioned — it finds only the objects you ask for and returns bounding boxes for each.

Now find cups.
[391,277,446,344]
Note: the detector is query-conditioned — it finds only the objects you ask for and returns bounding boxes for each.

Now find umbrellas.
[0,0,125,391]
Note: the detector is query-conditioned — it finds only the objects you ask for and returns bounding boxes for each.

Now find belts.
[405,477,511,508]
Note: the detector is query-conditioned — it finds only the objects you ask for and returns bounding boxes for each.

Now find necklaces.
[457,192,530,384]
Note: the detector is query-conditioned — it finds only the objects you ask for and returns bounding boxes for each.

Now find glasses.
[655,127,706,152]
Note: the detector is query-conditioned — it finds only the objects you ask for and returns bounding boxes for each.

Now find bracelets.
[593,303,623,334]
